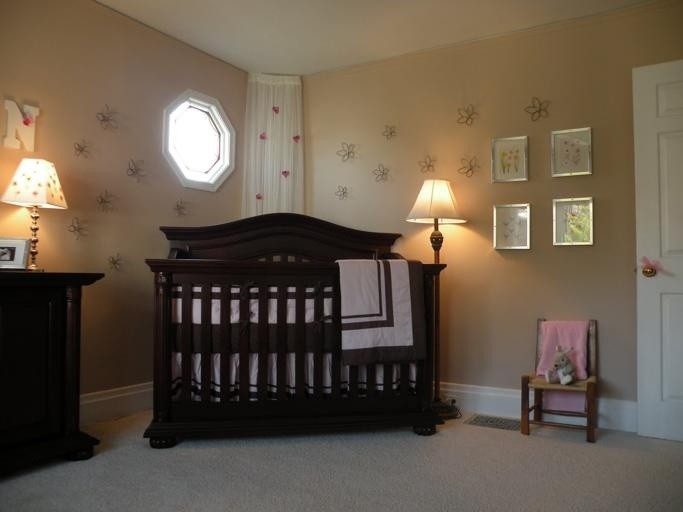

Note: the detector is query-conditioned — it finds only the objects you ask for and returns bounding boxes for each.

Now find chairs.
[520,319,600,442]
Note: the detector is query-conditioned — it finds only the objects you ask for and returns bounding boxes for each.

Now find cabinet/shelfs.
[0,273,105,479]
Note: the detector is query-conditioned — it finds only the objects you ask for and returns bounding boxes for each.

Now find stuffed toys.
[543,346,576,386]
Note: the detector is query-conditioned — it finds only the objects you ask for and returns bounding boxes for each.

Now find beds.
[143,213,447,448]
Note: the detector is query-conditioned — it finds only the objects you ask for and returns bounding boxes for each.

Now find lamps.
[406,180,469,419]
[0,158,68,271]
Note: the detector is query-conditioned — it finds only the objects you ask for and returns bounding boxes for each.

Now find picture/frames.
[491,135,529,183]
[551,127,593,177]
[493,203,531,250]
[0,237,31,269]
[552,197,594,246]
